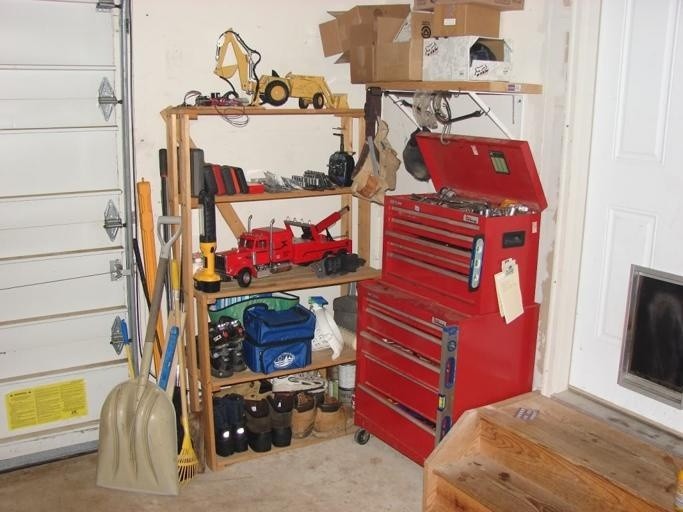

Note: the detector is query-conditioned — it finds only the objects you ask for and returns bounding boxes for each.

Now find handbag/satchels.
[351,87,401,205]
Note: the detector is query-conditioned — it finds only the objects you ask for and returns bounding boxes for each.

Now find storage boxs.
[318,0,525,84]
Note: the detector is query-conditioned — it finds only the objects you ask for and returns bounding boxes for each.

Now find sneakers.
[195,316,247,378]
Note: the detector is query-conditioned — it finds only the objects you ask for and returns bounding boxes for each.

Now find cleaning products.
[308,297,333,351]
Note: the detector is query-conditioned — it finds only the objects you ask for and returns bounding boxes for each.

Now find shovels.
[94,217,181,494]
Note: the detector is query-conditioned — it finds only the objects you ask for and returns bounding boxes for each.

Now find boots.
[214,390,359,457]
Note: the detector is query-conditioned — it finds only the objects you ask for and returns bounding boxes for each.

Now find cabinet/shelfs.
[165,105,382,471]
